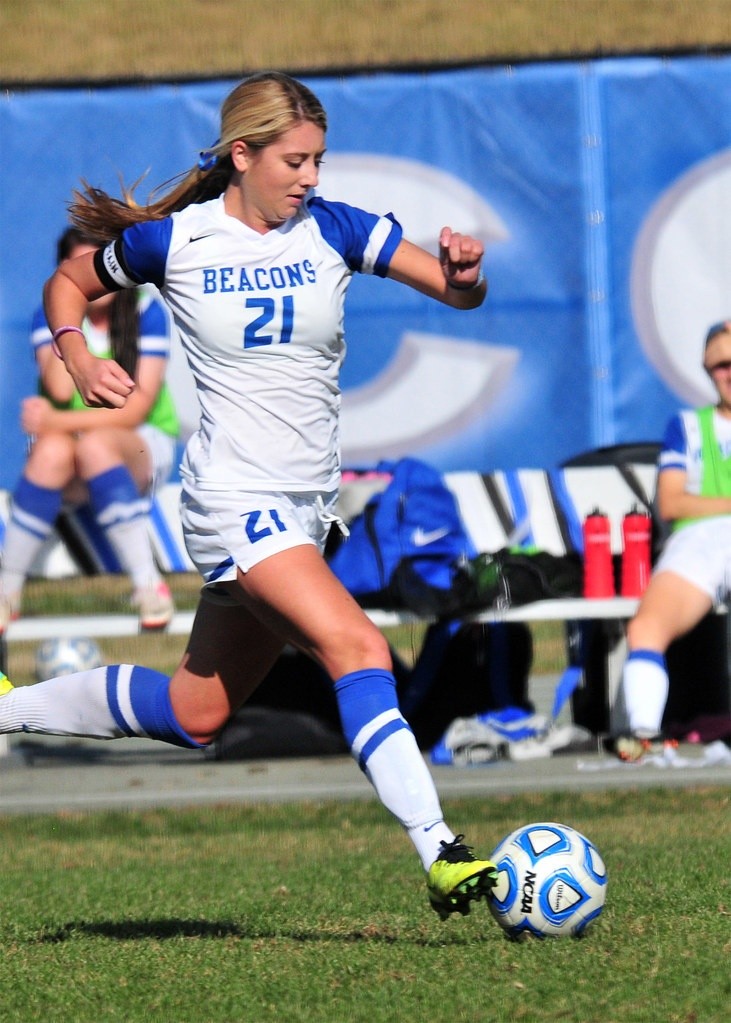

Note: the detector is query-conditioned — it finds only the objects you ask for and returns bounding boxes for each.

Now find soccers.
[484,821,608,943]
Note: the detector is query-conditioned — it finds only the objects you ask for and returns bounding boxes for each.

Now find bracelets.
[49,327,90,360]
[443,267,483,295]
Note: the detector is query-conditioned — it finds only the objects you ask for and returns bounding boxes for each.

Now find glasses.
[709,356,730,371]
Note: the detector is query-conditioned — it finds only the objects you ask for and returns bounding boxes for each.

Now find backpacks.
[326,455,478,608]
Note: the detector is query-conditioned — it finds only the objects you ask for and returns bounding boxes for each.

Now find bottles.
[621,501,653,597]
[582,507,615,597]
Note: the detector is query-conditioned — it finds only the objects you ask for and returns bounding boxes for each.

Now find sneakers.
[423,845,500,922]
[1,589,21,635]
[128,578,174,631]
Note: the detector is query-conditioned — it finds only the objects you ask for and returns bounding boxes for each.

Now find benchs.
[0,462,663,643]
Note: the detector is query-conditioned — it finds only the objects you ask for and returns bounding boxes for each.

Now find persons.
[600,321,730,765]
[1,74,496,920]
[2,219,177,633]
